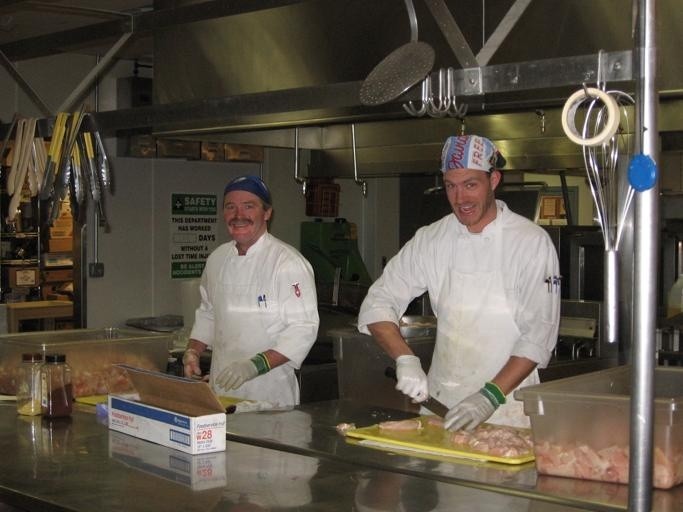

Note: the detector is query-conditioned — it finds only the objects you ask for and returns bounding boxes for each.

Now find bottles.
[39,351,73,420]
[14,351,41,417]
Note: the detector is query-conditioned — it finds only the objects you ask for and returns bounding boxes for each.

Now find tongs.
[2,110,113,223]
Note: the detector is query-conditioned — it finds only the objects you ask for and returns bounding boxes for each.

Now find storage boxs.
[324,314,450,420]
[513,365,683,492]
[0,327,175,403]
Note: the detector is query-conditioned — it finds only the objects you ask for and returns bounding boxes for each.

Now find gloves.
[395,355,429,404]
[215,352,272,392]
[444,381,507,432]
[181,348,203,379]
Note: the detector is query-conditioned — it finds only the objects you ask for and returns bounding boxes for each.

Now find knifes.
[383,365,474,432]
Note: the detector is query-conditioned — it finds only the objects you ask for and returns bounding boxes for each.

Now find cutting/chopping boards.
[344,412,535,467]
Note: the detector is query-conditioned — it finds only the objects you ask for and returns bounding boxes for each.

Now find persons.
[182,175,321,409]
[357,135,561,432]
